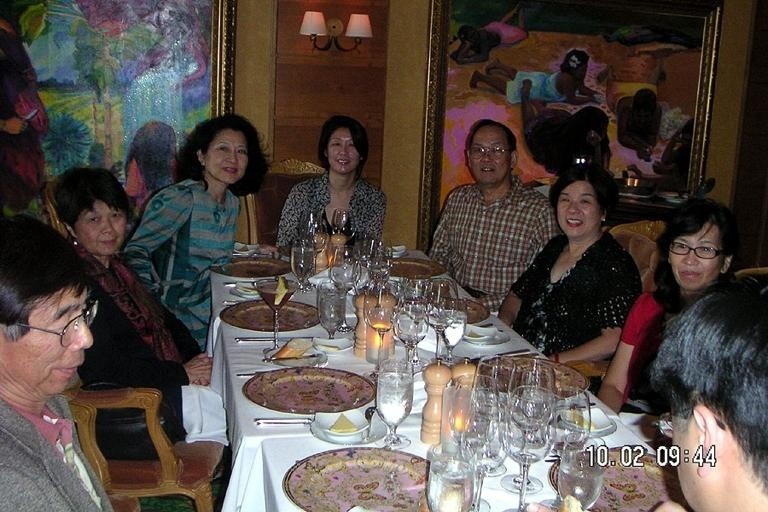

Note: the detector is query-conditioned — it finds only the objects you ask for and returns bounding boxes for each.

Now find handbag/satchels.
[80,381,173,462]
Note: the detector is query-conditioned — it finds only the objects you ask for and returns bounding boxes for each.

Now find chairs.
[548,217,668,381]
[42,179,212,336]
[229,158,327,247]
[63,360,229,512]
[625,266,768,438]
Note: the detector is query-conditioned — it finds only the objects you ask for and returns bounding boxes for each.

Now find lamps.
[298,11,376,52]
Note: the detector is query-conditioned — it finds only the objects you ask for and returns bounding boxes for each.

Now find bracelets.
[554,353,559,363]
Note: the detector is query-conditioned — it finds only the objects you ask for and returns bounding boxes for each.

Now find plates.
[311,410,372,436]
[559,408,614,432]
[228,282,260,300]
[309,420,388,445]
[399,293,492,325]
[220,299,321,332]
[468,331,511,345]
[480,354,591,399]
[281,446,474,512]
[242,367,376,416]
[266,349,330,369]
[387,256,449,279]
[313,336,355,353]
[461,323,499,343]
[549,449,695,512]
[551,412,619,438]
[208,256,293,278]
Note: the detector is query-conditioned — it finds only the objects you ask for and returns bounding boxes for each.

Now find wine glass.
[374,357,415,450]
[366,260,391,304]
[360,278,402,359]
[317,280,346,339]
[256,278,301,354]
[330,247,362,332]
[391,278,468,371]
[438,356,559,512]
[290,238,394,293]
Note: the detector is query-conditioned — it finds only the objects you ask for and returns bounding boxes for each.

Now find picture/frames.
[0,0,235,230]
[415,2,724,254]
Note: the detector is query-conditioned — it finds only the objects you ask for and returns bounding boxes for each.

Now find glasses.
[670,241,726,259]
[468,144,511,160]
[16,299,99,348]
[651,407,724,440]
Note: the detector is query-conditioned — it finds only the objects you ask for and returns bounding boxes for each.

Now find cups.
[557,434,609,511]
[548,389,591,460]
[428,446,478,512]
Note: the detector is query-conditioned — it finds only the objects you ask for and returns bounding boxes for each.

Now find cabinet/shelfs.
[604,192,713,244]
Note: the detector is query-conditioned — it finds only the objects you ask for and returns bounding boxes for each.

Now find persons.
[121,114,278,350]
[0,19,49,224]
[429,119,562,318]
[275,115,387,251]
[121,121,178,223]
[1,213,116,512]
[498,168,643,398]
[598,197,735,445]
[52,166,230,446]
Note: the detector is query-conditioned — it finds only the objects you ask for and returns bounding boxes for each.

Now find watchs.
[651,277,767,512]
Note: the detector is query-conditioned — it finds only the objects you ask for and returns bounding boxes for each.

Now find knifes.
[232,335,321,342]
